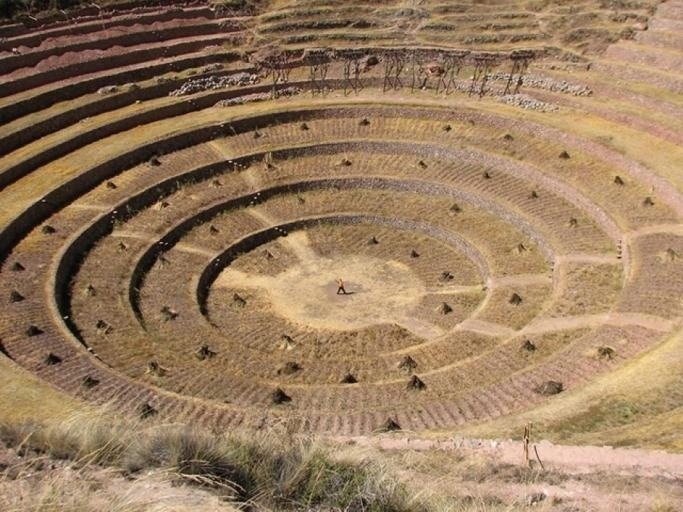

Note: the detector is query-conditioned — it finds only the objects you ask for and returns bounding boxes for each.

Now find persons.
[335,278,347,294]
